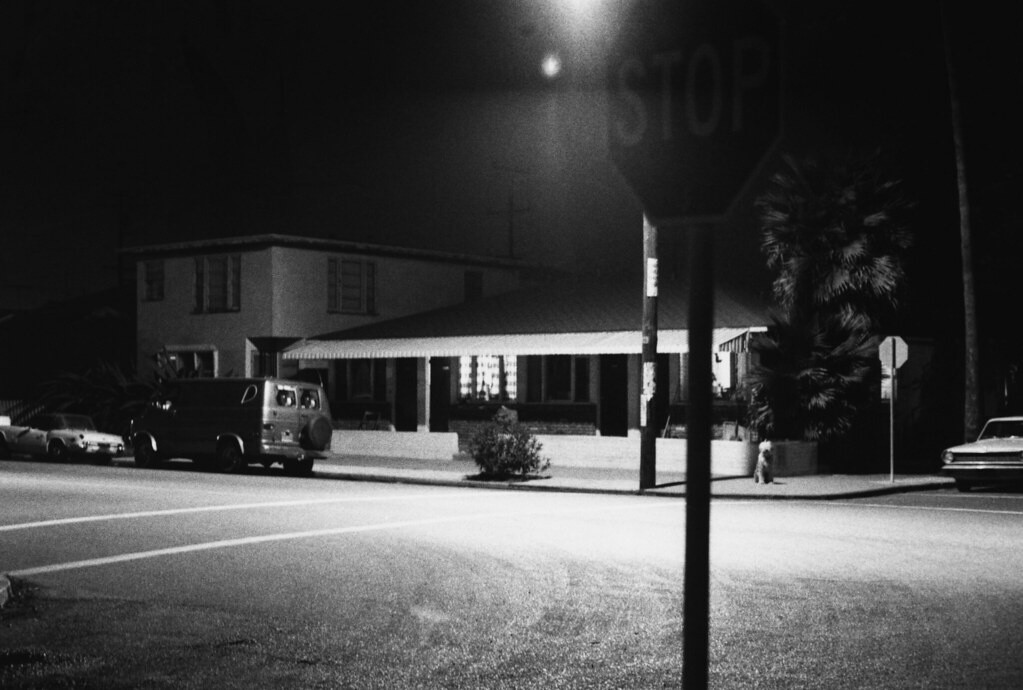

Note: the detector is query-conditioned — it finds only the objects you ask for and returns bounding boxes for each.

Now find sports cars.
[0,410,125,462]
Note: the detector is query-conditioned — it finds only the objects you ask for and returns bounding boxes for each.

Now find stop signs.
[597,0,791,231]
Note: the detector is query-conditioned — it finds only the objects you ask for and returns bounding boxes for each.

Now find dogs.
[754,441,775,484]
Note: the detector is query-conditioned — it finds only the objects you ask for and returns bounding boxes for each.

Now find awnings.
[283,326,751,360]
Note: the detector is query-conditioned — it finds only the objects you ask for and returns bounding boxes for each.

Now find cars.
[940,414,1023,492]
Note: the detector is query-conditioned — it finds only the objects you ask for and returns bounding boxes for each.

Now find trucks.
[126,376,334,475]
